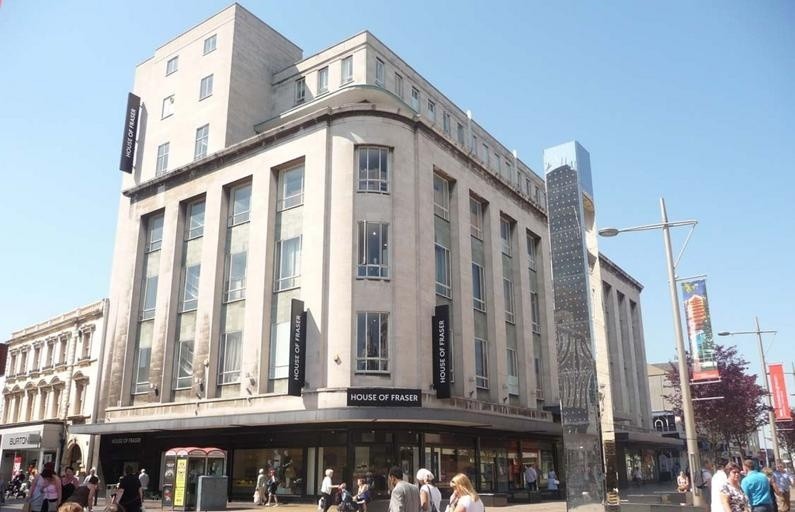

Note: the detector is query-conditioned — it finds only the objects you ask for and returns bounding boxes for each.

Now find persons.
[525,465,537,491]
[388,467,419,512]
[256,469,267,505]
[267,460,273,476]
[548,468,558,496]
[321,469,338,512]
[282,450,295,488]
[417,468,442,512]
[273,449,281,469]
[265,470,278,507]
[357,479,368,512]
[634,467,643,486]
[13,463,150,512]
[449,473,484,512]
[677,460,795,512]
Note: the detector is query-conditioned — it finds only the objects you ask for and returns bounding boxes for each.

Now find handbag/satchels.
[48,501,58,512]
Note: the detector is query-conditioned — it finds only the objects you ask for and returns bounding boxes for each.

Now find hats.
[416,467,435,481]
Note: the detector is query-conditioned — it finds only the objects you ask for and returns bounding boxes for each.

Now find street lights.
[593,196,706,507]
[716,315,782,471]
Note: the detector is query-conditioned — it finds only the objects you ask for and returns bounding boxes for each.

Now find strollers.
[7,481,25,499]
[339,488,362,512]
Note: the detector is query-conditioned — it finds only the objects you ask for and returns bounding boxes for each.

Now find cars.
[782,460,793,472]
